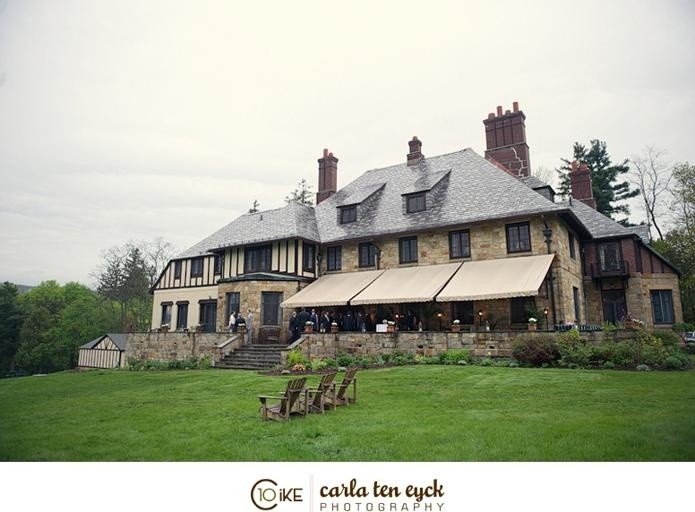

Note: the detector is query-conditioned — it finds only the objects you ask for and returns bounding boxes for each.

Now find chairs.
[256,364,359,424]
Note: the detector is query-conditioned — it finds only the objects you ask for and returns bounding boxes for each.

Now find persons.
[234,312,245,330]
[288,307,417,346]
[229,311,236,331]
[246,308,254,344]
